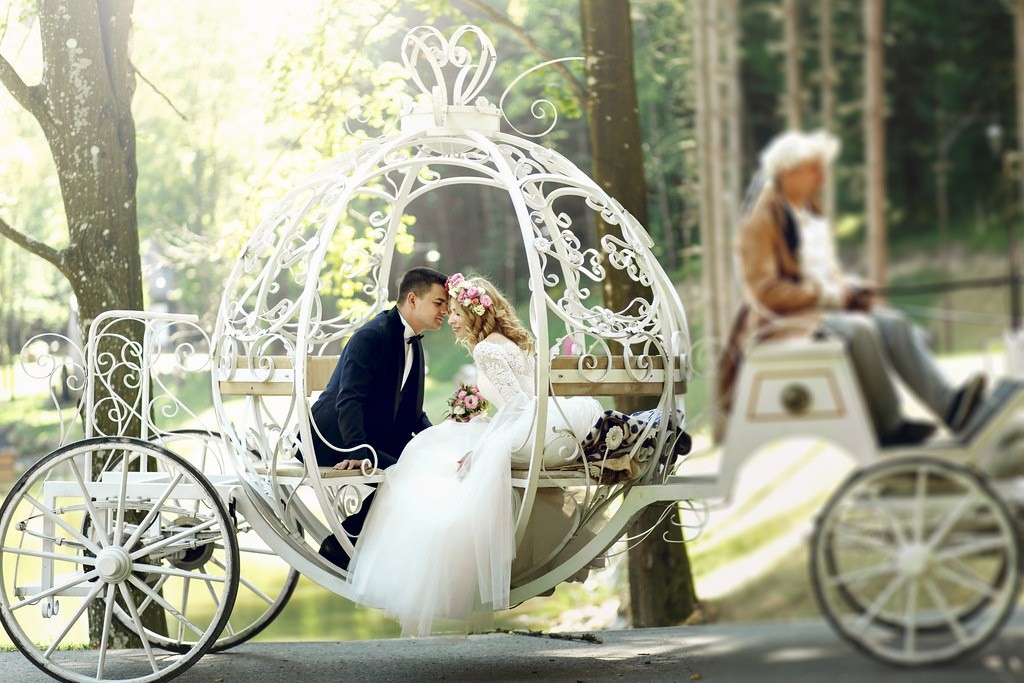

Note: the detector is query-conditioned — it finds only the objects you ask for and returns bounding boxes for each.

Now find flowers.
[448,381,492,424]
[442,272,493,318]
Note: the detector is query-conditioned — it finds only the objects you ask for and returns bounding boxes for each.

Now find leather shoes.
[317,536,346,570]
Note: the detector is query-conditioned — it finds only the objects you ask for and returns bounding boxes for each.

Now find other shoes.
[879,373,988,446]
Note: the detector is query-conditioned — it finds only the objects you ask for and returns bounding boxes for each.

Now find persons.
[345,275,617,637]
[292,266,450,573]
[741,128,986,449]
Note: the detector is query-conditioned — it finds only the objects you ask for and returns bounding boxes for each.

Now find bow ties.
[407,334,424,345]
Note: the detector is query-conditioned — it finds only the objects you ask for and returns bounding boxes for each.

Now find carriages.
[0,21,1024,682]
